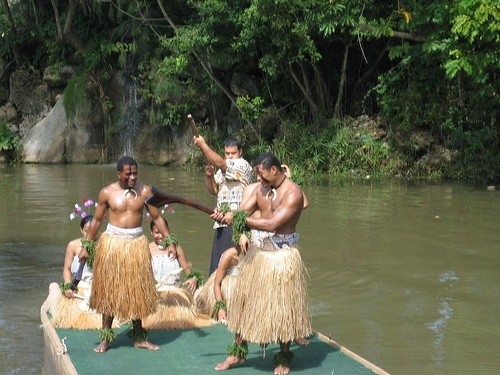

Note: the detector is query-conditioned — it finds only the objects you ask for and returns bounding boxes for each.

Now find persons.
[196,247,241,325]
[240,165,310,348]
[142,216,218,331]
[194,135,254,277]
[78,157,177,352]
[210,152,312,375]
[51,216,121,329]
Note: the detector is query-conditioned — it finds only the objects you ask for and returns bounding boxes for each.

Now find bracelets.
[80,239,94,248]
[61,283,75,293]
[232,209,248,232]
[185,272,203,286]
[220,204,231,212]
[232,229,252,245]
[212,300,225,319]
[162,233,178,248]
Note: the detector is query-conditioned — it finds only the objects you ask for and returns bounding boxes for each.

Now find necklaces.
[119,183,137,200]
[266,174,286,201]
[158,244,166,249]
